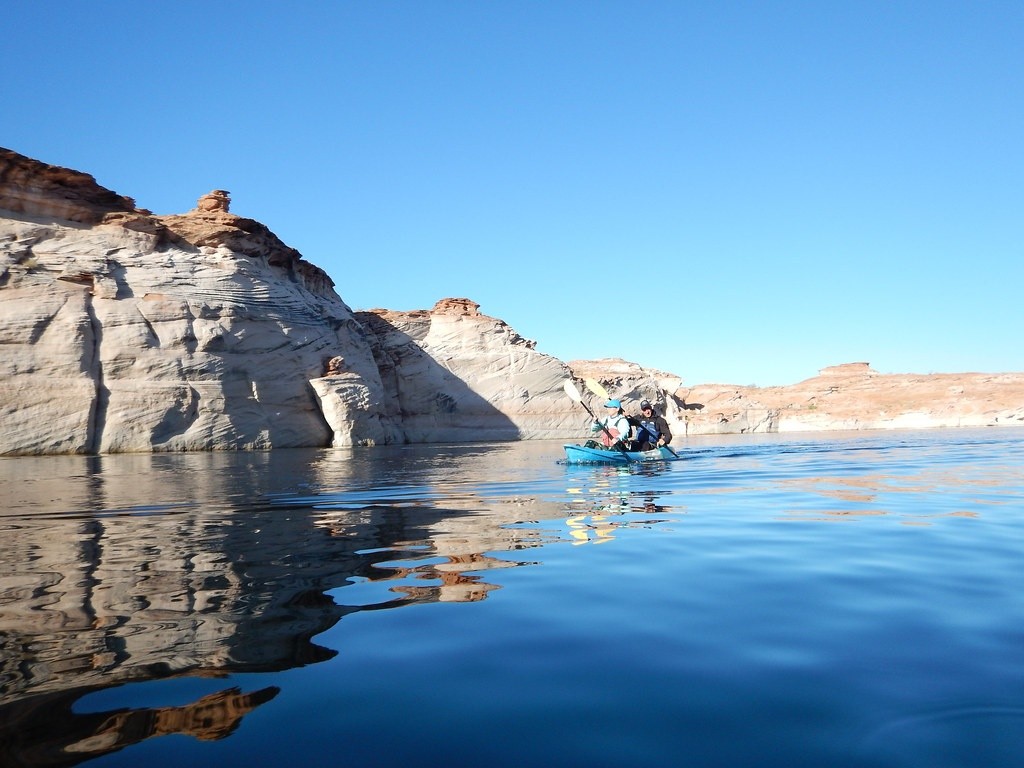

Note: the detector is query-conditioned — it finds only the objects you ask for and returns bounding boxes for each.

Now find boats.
[564,443,676,465]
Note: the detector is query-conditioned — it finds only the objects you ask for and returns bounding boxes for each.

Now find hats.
[641,400,650,409]
[604,399,620,409]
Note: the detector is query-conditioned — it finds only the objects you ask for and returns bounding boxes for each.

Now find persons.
[623,400,672,451]
[576,400,631,452]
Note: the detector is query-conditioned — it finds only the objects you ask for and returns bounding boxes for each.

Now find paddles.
[564,379,631,461]
[586,379,679,458]
